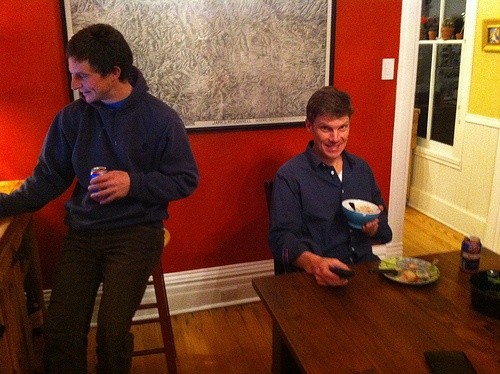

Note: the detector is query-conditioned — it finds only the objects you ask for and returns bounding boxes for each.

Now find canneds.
[91,167,108,202]
[459,235,482,273]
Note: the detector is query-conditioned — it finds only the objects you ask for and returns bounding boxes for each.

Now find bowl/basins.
[342,198,381,228]
[469,269,500,318]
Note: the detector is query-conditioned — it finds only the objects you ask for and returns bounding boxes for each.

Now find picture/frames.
[60,0,336,131]
[482,19,500,52]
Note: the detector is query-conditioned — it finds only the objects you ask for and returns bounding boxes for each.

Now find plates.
[379,257,441,286]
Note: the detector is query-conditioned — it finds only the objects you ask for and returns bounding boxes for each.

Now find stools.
[131,227,180,374]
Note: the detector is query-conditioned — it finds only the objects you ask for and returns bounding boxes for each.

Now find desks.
[252,245,500,374]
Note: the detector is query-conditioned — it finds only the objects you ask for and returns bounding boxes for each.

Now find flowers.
[421,15,437,29]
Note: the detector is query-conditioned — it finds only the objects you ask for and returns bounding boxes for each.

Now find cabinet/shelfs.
[0,180,46,374]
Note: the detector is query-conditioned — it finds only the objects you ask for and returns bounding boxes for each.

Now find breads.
[400,269,417,282]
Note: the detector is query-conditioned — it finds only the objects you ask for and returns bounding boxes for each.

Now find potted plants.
[441,13,464,40]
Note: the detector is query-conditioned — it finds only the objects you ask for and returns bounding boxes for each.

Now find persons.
[1,24,199,373]
[268,86,393,287]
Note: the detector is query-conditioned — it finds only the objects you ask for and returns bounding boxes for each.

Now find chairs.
[264,180,285,276]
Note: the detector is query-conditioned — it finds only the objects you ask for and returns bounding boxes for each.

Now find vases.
[427,30,438,40]
